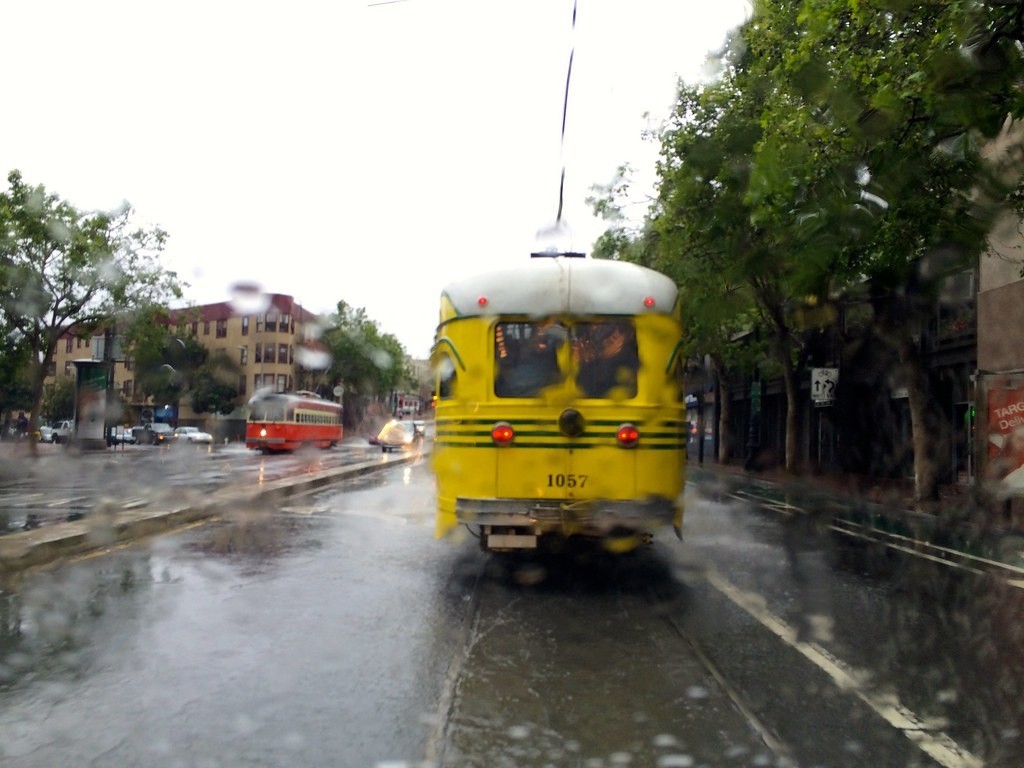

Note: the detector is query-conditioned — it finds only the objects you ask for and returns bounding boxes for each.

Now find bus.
[246,391,343,455]
[432,235,694,574]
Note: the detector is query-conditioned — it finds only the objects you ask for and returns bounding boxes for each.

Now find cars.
[368,419,426,452]
[3,413,215,447]
